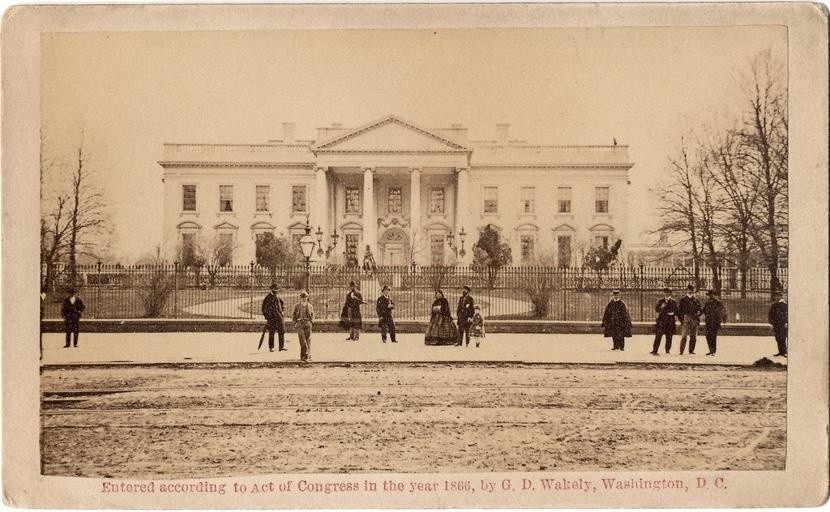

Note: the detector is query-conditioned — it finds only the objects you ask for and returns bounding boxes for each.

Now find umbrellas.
[257,323,268,351]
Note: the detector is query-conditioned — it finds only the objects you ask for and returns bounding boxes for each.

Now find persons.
[467,305,485,347]
[339,281,363,341]
[600,289,633,351]
[261,282,287,352]
[375,284,399,344]
[649,288,678,355]
[701,289,728,356]
[360,244,374,276]
[768,289,787,358]
[674,284,704,355]
[424,289,458,346]
[60,287,86,348]
[454,285,475,347]
[292,291,316,361]
